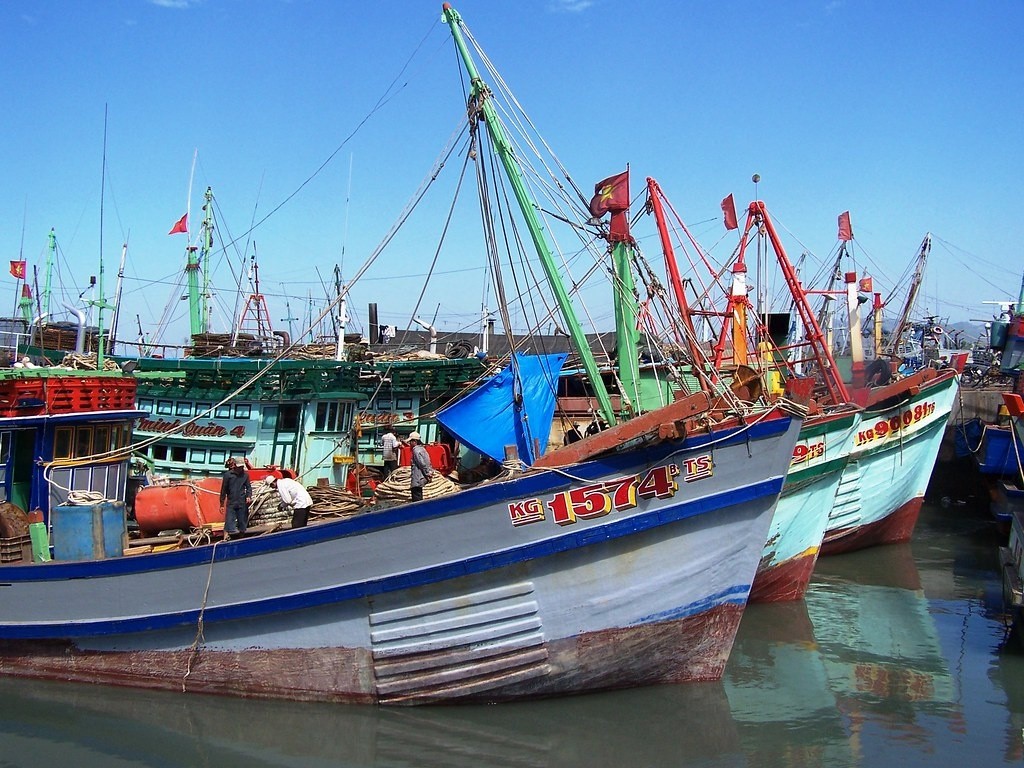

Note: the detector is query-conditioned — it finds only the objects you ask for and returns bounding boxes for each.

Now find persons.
[568,422,583,444]
[219,458,253,539]
[992,349,1002,368]
[407,435,433,503]
[382,430,403,479]
[265,475,314,529]
[584,413,608,438]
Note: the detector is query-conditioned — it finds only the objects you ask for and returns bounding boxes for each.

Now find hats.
[573,421,580,426]
[266,475,275,488]
[392,427,399,435]
[225,458,236,468]
[404,431,421,441]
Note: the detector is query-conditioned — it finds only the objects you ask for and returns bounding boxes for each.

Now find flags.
[858,278,872,291]
[721,193,738,231]
[590,169,630,218]
[10,260,25,279]
[22,284,32,298]
[169,214,187,236]
[837,210,852,241]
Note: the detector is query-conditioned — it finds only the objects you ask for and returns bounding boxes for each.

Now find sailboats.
[0,1,1024,714]
